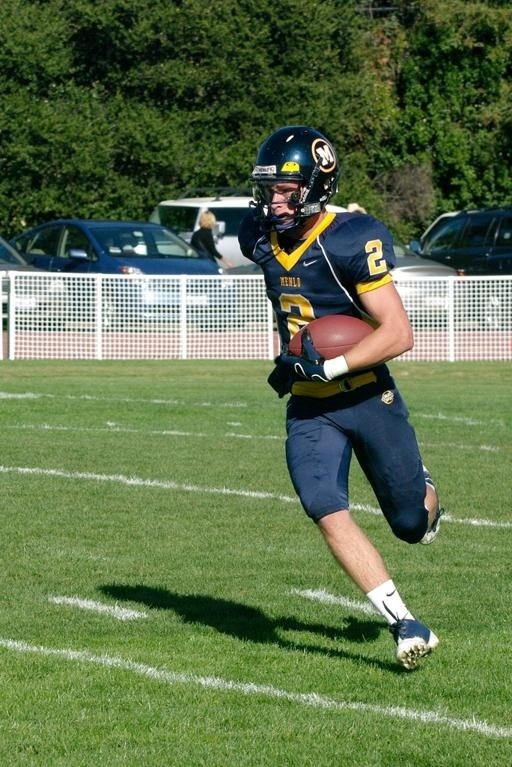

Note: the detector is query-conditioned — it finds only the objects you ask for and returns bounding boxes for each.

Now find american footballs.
[288,315,375,360]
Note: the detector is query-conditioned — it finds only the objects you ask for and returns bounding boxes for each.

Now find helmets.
[247,125,341,235]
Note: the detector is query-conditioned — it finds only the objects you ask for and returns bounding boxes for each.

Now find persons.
[190,210,234,270]
[237,126,444,673]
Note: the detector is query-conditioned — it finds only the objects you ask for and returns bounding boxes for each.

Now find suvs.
[410,206,512,332]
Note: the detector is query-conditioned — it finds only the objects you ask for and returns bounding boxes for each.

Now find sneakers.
[388,616,440,671]
[419,464,445,546]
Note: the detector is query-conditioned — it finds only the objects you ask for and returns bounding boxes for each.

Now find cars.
[387,239,469,330]
[0,235,72,333]
[5,216,236,333]
[412,207,478,244]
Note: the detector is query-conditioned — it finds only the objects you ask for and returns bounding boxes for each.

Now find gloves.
[278,328,331,384]
[268,357,292,398]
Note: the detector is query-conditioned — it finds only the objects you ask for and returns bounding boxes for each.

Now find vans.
[148,186,352,269]
